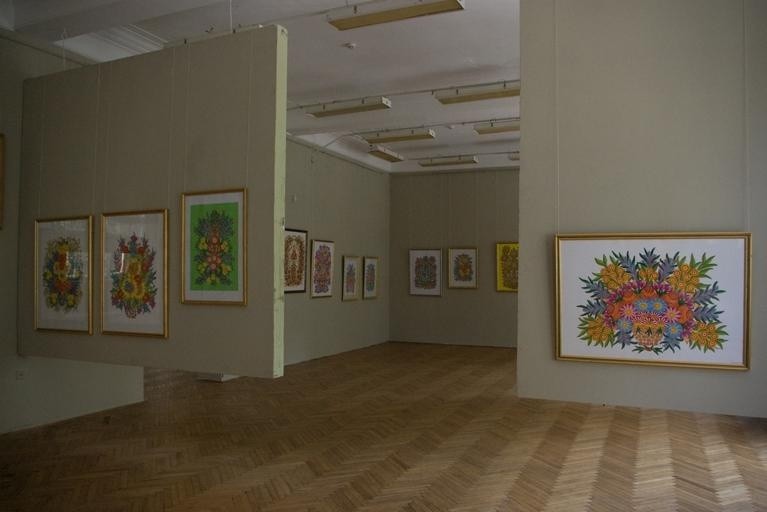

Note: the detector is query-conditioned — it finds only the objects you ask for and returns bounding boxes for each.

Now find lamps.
[433,78,522,105]
[362,124,437,144]
[417,153,482,168]
[160,1,264,50]
[471,115,519,135]
[365,142,405,166]
[323,0,468,32]
[308,94,394,118]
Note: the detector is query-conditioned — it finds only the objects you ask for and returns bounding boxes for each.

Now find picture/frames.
[495,241,518,292]
[342,253,362,301]
[179,187,247,306]
[447,246,479,289]
[310,238,335,298]
[363,254,379,301]
[284,226,308,294]
[98,209,170,337]
[408,247,442,298]
[32,215,92,335]
[554,230,753,371]
